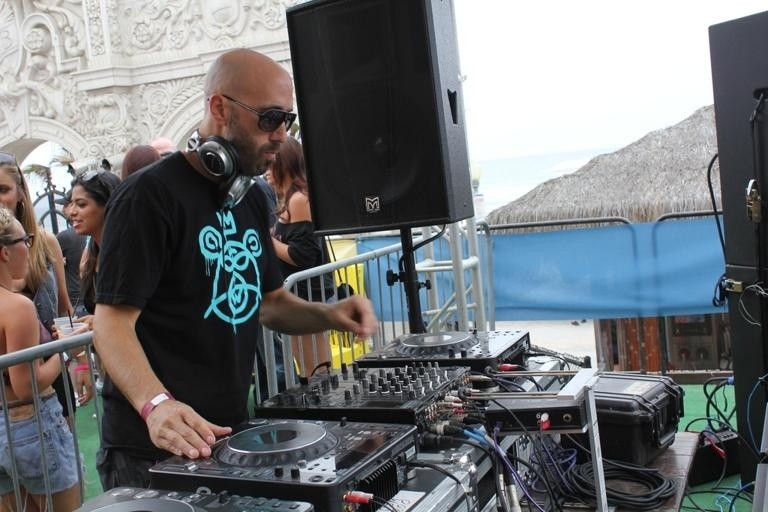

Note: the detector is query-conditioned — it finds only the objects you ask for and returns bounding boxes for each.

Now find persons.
[122,137,175,180]
[257,135,338,398]
[90,48,376,490]
[0,152,122,510]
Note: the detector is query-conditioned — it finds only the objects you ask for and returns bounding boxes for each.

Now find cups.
[54,316,88,336]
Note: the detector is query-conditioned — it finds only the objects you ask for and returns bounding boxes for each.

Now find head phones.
[186,129,256,209]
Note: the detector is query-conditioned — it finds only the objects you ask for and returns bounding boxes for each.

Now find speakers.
[286,0,474,235]
[708,11,768,270]
[725,266,768,493]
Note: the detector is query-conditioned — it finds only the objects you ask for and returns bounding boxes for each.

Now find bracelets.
[140,392,175,419]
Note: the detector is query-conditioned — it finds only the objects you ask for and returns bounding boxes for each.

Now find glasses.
[4,233,34,248]
[1,152,16,162]
[222,95,296,131]
[81,171,110,196]
